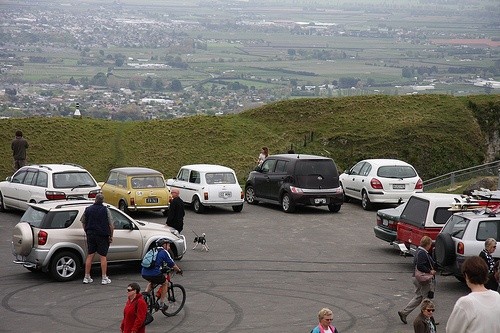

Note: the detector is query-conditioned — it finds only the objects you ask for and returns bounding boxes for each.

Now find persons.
[11,130,29,174]
[141,238,183,310]
[445,237,500,333]
[120,282,148,333]
[397,236,440,325]
[80,193,113,284]
[412,300,438,333]
[161,188,186,233]
[309,306,339,333]
[257,147,270,168]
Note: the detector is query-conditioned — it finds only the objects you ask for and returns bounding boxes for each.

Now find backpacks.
[141,247,163,269]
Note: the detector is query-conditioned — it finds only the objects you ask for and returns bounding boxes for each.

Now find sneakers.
[398,311,408,324]
[83,277,94,283]
[101,277,111,284]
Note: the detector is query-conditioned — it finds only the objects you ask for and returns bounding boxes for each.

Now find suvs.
[393,193,500,288]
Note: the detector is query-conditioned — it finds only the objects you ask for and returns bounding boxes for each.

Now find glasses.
[128,289,134,292]
[426,309,435,312]
[490,246,496,248]
[323,319,332,322]
[171,192,175,194]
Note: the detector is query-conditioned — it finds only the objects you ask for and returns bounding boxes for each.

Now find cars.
[11,198,187,282]
[374,202,408,242]
[98,167,172,217]
[339,159,423,211]
[245,154,344,213]
[165,164,245,214]
[0,164,102,213]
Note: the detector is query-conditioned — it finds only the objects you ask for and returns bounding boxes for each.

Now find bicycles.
[140,265,186,317]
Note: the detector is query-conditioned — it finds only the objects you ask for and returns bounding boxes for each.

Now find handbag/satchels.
[415,268,433,285]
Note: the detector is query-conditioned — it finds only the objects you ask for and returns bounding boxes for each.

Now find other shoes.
[158,304,167,310]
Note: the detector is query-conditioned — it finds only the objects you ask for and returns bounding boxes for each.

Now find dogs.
[192,233,210,252]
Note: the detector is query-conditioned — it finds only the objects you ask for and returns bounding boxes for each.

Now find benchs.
[56,174,81,185]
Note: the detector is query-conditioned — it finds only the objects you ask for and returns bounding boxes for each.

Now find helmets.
[156,238,171,246]
